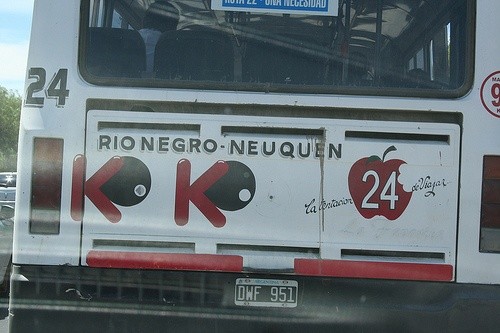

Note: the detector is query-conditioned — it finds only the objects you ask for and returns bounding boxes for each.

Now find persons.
[133,0,180,79]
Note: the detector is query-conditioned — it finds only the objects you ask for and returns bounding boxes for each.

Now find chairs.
[243,20,325,86]
[86,27,148,77]
[153,25,234,82]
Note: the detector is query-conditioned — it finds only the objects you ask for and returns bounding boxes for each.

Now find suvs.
[0,172,16,203]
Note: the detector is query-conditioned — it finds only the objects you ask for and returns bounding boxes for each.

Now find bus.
[9,0,500,333]
